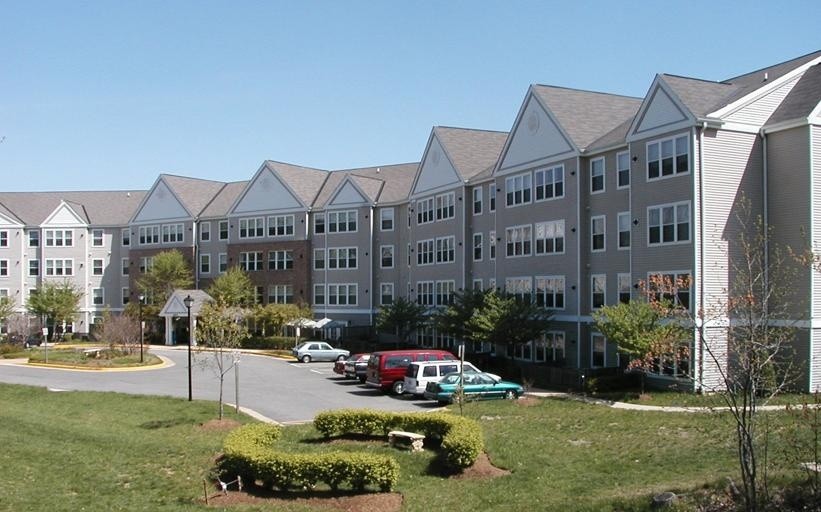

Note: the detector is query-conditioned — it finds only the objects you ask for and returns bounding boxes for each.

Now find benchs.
[387,427,428,454]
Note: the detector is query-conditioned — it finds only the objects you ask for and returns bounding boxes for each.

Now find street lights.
[136,293,147,363]
[183,295,195,401]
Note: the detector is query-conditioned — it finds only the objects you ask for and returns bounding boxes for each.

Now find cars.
[293,339,525,404]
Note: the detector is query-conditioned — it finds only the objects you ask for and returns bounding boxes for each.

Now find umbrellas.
[286,317,315,337]
